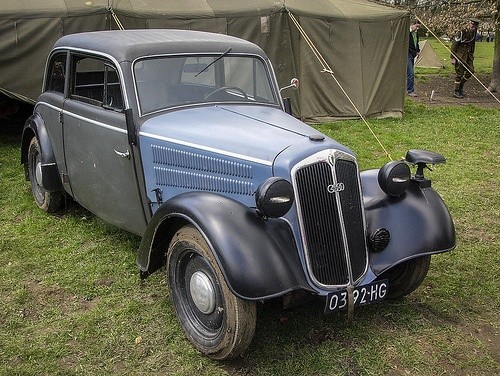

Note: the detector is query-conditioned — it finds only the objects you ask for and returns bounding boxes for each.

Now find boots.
[453,82,463,99]
[460,76,468,91]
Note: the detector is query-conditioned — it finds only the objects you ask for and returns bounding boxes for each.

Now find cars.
[21,29,458,361]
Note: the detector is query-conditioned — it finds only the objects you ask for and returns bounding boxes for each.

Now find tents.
[0,0,411,120]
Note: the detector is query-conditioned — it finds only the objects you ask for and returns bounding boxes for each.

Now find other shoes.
[407,92,417,97]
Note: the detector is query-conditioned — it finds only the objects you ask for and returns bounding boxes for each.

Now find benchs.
[76,71,125,110]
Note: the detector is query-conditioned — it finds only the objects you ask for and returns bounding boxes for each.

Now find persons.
[407,20,422,97]
[450,18,481,99]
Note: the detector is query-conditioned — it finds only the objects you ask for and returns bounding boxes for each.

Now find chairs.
[135,73,170,111]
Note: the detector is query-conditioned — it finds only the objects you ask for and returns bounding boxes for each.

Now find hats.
[470,18,480,24]
